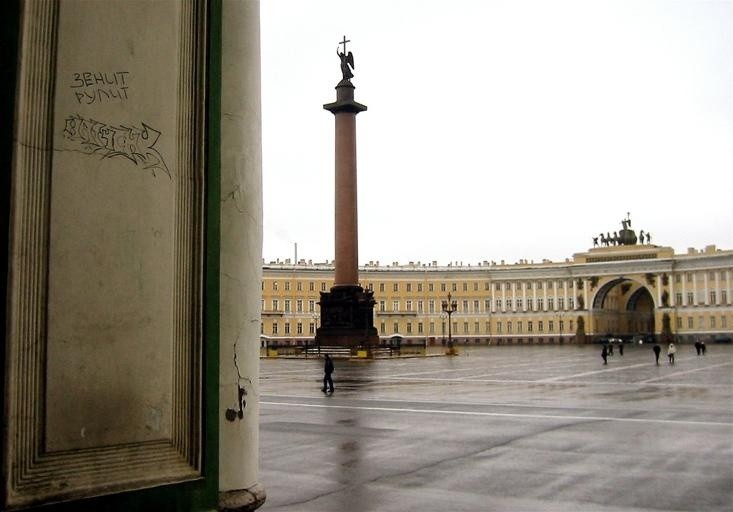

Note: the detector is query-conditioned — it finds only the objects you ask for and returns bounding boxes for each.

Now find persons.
[695,340,701,355]
[336,47,354,81]
[601,342,624,365]
[646,232,650,244]
[321,354,334,393]
[653,343,660,365]
[668,342,676,364]
[593,237,599,248]
[700,341,705,355]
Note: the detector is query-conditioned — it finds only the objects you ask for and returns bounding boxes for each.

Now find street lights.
[441,291,457,341]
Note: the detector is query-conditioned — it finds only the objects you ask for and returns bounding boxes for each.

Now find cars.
[590,330,654,345]
